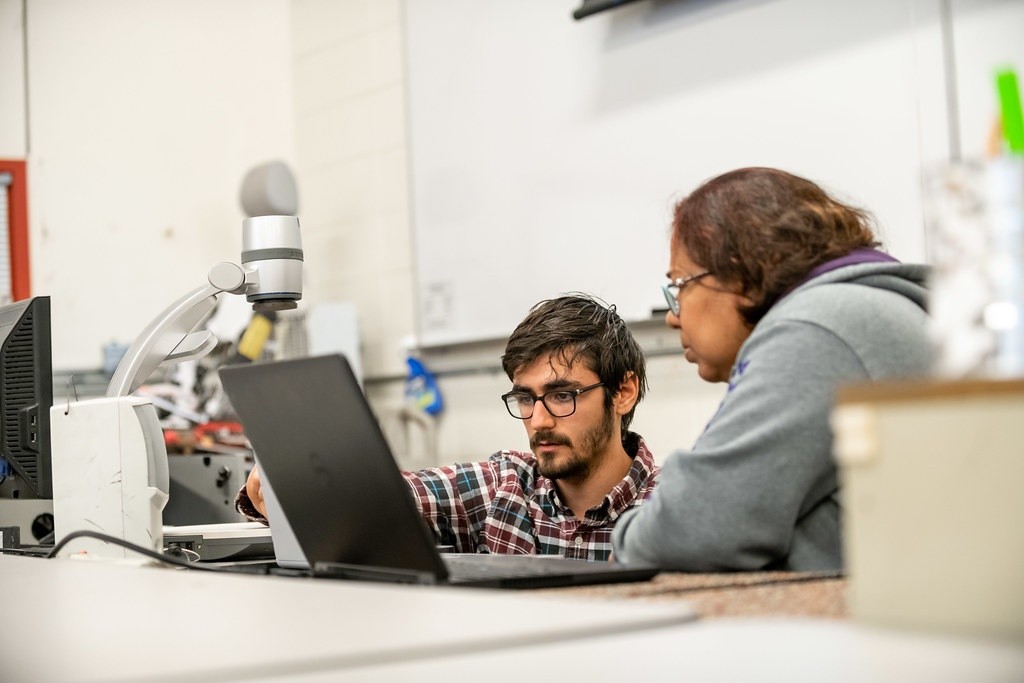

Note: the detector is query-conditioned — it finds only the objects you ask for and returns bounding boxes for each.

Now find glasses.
[502,383,604,418]
[663,271,714,315]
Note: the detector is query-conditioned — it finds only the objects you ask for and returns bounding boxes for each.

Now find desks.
[0,525,1024,683]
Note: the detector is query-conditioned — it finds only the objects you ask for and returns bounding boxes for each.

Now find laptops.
[215,353,661,588]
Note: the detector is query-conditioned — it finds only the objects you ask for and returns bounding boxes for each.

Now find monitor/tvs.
[0,295,55,556]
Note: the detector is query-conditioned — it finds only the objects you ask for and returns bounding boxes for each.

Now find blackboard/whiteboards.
[396,1,965,359]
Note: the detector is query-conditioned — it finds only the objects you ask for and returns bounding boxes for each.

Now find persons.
[235,291,658,561]
[609,166,944,571]
